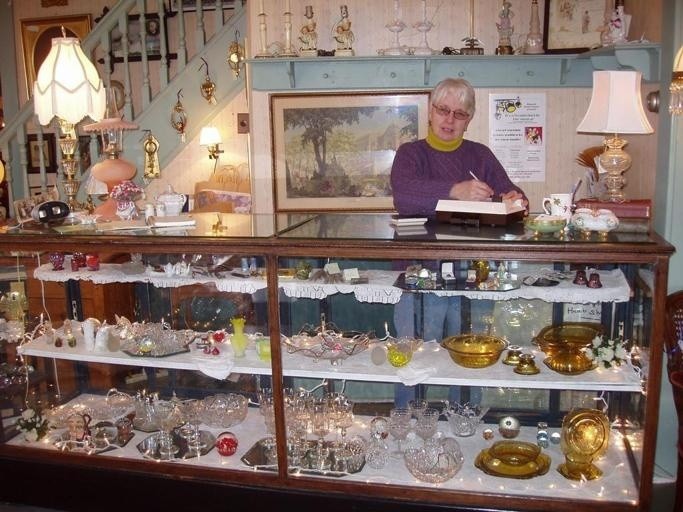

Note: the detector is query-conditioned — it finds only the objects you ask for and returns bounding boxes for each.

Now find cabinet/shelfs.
[0,210,327,512]
[263,210,675,512]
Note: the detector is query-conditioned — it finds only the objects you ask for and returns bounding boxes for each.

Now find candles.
[258,0,264,14]
[284,0,290,12]
[468,0,474,40]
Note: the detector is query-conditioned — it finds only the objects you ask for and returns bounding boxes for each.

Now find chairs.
[664,290,682,488]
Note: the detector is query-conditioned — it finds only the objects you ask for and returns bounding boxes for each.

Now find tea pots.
[157,185,186,216]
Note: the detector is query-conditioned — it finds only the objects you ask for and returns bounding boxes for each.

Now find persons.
[393,78,529,415]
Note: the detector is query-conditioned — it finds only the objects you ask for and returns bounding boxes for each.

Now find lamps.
[574,67,655,203]
[81,86,140,221]
[195,124,227,162]
[31,24,108,220]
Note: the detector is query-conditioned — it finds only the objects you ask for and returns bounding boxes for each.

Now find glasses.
[432,103,469,120]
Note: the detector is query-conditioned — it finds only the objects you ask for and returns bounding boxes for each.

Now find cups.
[385,338,415,367]
[258,336,271,362]
[542,193,572,223]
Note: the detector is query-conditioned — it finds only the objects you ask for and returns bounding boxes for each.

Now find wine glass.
[386,398,441,464]
[254,385,356,472]
[150,398,204,454]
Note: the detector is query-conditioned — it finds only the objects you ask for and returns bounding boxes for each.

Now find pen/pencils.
[470,171,481,182]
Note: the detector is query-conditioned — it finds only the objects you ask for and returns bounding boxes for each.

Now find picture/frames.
[17,12,93,102]
[92,6,178,64]
[24,132,57,173]
[168,0,246,14]
[542,0,622,53]
[266,89,432,214]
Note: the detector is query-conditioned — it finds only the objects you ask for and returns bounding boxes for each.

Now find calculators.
[390,215,428,226]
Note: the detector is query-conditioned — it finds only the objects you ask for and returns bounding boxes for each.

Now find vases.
[227,317,248,359]
[116,202,140,221]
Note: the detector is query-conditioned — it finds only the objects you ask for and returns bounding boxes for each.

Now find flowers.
[108,178,144,204]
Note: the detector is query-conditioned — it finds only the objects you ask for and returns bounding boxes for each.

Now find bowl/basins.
[489,439,542,465]
[198,391,249,428]
[279,313,371,363]
[439,334,507,369]
[442,403,488,438]
[532,321,601,374]
[523,215,567,238]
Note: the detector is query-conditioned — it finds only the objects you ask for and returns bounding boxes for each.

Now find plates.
[473,446,551,479]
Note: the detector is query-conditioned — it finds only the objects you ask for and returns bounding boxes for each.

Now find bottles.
[45,319,55,344]
[63,318,71,335]
[81,319,94,351]
[364,433,388,468]
[144,203,154,222]
[156,202,165,217]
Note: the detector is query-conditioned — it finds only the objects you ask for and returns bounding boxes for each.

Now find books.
[391,211,427,226]
[395,227,428,237]
[146,215,195,229]
